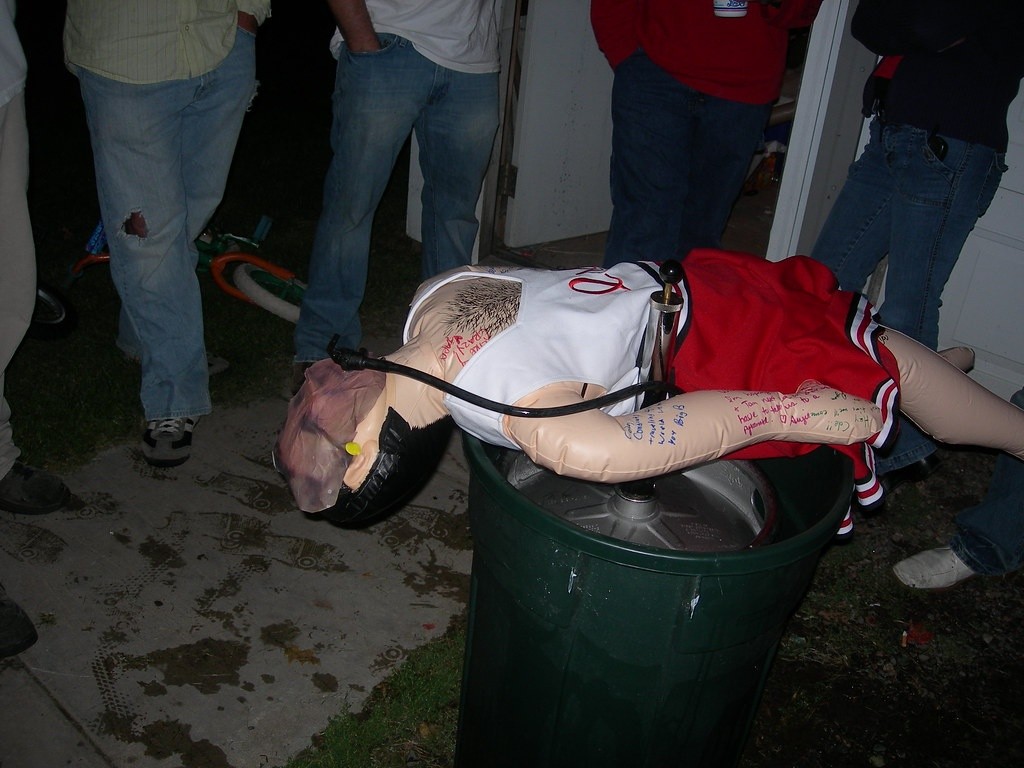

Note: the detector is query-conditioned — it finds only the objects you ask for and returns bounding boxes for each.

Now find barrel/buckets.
[453,435,854,767]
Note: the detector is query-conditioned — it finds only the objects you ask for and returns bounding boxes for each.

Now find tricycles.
[25,211,308,341]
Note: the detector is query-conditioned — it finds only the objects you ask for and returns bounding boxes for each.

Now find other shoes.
[290,361,314,397]
[878,449,944,496]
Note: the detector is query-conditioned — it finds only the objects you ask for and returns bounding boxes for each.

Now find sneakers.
[0,458,70,514]
[102,354,136,419]
[142,415,202,468]
[0,582,38,657]
[892,544,985,594]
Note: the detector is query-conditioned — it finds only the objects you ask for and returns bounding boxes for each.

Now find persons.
[892,472,1024,588]
[292,1,506,396]
[275,248,1024,529]
[63,0,272,465]
[807,1,1024,351]
[590,0,822,270]
[0,0,71,653]
